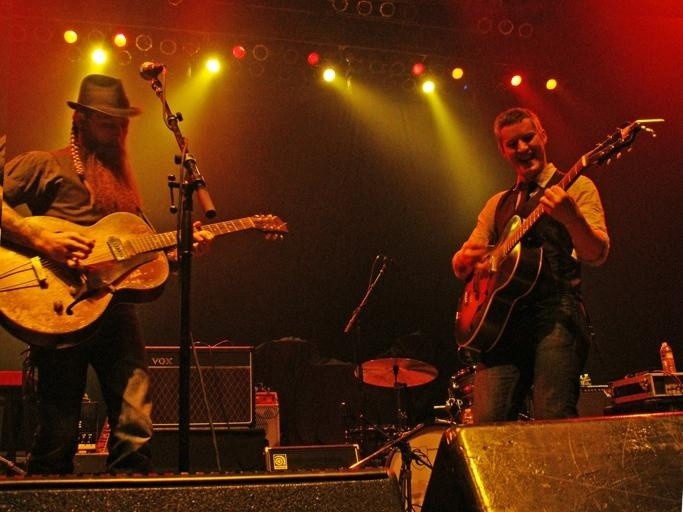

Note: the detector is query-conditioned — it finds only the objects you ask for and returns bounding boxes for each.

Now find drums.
[443,365,481,427]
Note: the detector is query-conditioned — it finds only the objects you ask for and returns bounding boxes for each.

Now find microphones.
[138,61,166,79]
[377,253,398,270]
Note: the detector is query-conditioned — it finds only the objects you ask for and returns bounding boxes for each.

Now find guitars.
[0,211,288,350]
[452,118,667,353]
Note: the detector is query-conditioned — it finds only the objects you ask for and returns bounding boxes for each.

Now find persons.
[451,107,612,425]
[0,73,214,476]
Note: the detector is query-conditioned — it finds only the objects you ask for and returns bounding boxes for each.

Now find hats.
[65,73,143,120]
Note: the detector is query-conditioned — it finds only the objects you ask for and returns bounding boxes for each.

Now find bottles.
[660,342,674,373]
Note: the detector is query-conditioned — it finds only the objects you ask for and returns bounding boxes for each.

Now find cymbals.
[354,355,438,388]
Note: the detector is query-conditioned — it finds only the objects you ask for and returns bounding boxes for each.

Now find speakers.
[145,344,254,426]
[2,470,404,511]
[419,412,682,511]
